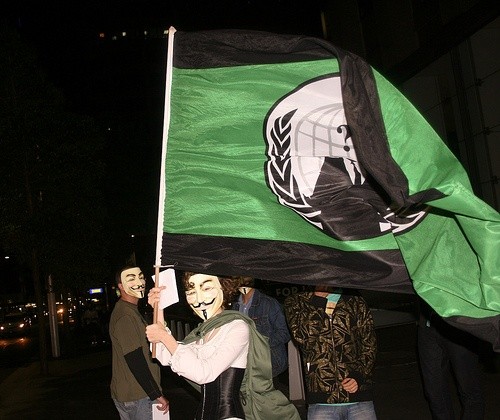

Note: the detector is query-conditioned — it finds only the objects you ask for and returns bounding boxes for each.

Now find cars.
[0,311,31,339]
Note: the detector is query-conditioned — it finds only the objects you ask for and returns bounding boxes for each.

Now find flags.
[157,29,500,353]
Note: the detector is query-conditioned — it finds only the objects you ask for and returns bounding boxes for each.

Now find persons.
[284,284,378,420]
[231,279,290,392]
[416,314,485,420]
[146,272,301,420]
[84,302,113,344]
[109,265,168,420]
